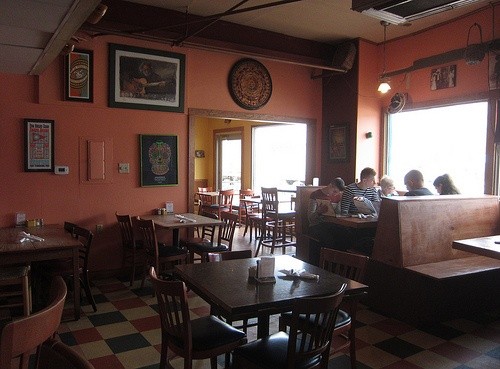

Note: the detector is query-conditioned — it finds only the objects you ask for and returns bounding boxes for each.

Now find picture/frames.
[139,133,180,188]
[23,118,55,172]
[64,47,94,103]
[108,41,185,113]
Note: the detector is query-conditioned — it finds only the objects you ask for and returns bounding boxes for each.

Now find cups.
[313,177,319,186]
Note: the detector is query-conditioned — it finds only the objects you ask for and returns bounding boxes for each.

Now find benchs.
[295,185,500,326]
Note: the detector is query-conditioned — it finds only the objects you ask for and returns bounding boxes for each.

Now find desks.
[0,224,84,319]
[240,197,292,203]
[323,214,379,228]
[172,254,368,339]
[138,212,225,250]
[452,235,500,261]
[196,192,240,196]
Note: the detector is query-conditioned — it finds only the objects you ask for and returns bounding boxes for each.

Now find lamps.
[378,22,391,93]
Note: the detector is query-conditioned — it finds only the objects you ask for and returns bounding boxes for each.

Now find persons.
[434,174,462,195]
[307,166,400,250]
[403,170,434,197]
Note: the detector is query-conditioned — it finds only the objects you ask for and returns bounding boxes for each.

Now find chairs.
[0,187,372,369]
